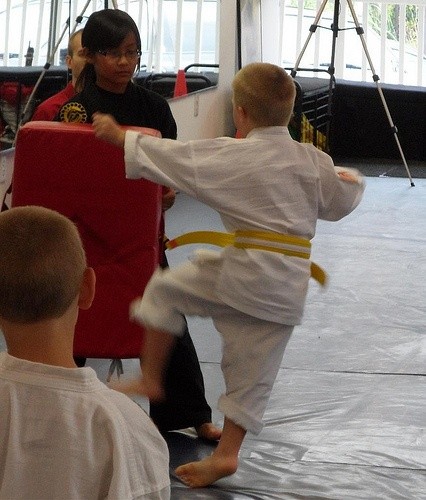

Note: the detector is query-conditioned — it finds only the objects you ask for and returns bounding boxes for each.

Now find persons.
[30,9,224,441]
[0,205,171,500]
[92,62,367,488]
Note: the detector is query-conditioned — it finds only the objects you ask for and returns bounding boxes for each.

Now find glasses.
[94,47,141,57]
